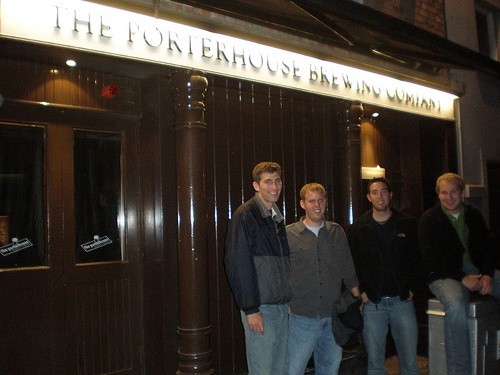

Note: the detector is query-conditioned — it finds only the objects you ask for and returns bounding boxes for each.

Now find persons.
[285,183,360,375]
[348,177,427,375]
[418,172,500,375]
[224,161,293,375]
